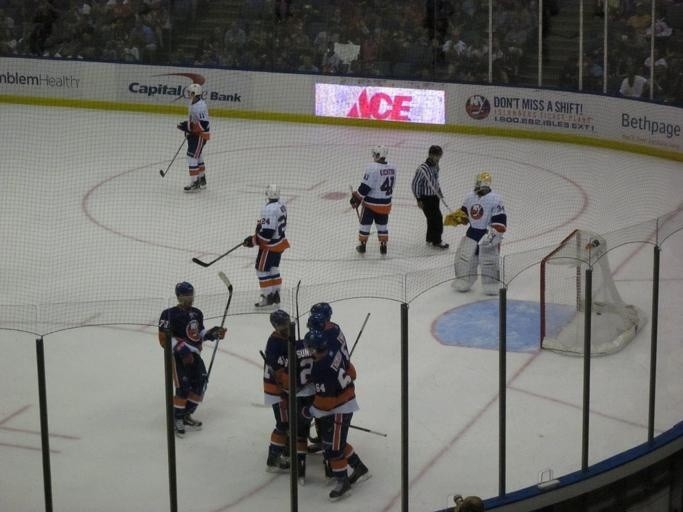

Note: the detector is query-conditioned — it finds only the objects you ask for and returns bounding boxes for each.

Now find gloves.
[174,342,193,365]
[177,121,187,131]
[350,192,362,209]
[243,236,253,247]
[207,327,226,340]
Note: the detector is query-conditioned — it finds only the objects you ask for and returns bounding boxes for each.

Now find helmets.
[311,302,332,322]
[372,146,388,161]
[270,311,290,333]
[308,314,326,332]
[184,84,203,98]
[429,146,442,161]
[304,331,329,353]
[265,184,280,199]
[176,281,194,297]
[473,172,491,192]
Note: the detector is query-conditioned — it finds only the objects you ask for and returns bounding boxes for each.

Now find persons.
[444,168,506,295]
[298,330,371,501]
[2,0,683,110]
[175,84,209,191]
[242,184,289,310]
[410,145,450,248]
[157,281,226,438]
[293,314,334,479]
[310,303,348,353]
[262,309,298,470]
[454,494,484,512]
[349,144,396,255]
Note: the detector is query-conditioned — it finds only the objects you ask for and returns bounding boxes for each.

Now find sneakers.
[325,462,335,478]
[267,458,290,469]
[329,481,350,498]
[379,243,387,254]
[355,245,366,253]
[199,178,205,185]
[349,466,368,484]
[298,460,306,477]
[272,291,280,303]
[185,182,199,190]
[433,240,449,248]
[175,416,185,433]
[426,237,431,242]
[255,294,272,307]
[308,442,322,453]
[185,413,202,426]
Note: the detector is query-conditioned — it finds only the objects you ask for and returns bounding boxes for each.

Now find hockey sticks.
[192,241,245,267]
[160,136,187,176]
[259,350,321,443]
[351,184,377,235]
[203,272,232,391]
